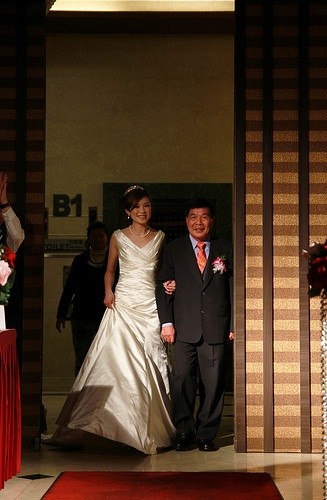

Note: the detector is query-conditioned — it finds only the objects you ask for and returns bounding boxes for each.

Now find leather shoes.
[199,438,215,451]
[175,434,196,450]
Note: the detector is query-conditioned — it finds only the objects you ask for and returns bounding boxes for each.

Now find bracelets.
[0,202,10,210]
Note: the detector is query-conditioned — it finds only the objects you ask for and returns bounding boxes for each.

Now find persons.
[80,185,177,451]
[55,221,120,375]
[155,200,235,453]
[0,172,28,255]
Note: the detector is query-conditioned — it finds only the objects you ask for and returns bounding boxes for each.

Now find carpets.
[41,470,283,500]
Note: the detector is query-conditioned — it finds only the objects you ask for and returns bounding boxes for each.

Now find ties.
[196,241,207,275]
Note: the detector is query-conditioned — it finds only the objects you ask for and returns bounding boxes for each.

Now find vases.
[0,305,6,331]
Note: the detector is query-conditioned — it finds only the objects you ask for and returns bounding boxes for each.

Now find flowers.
[211,254,227,275]
[0,235,16,304]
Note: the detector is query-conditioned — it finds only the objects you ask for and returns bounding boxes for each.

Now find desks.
[0,328,21,490]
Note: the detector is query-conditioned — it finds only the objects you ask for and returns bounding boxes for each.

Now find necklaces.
[129,225,152,238]
